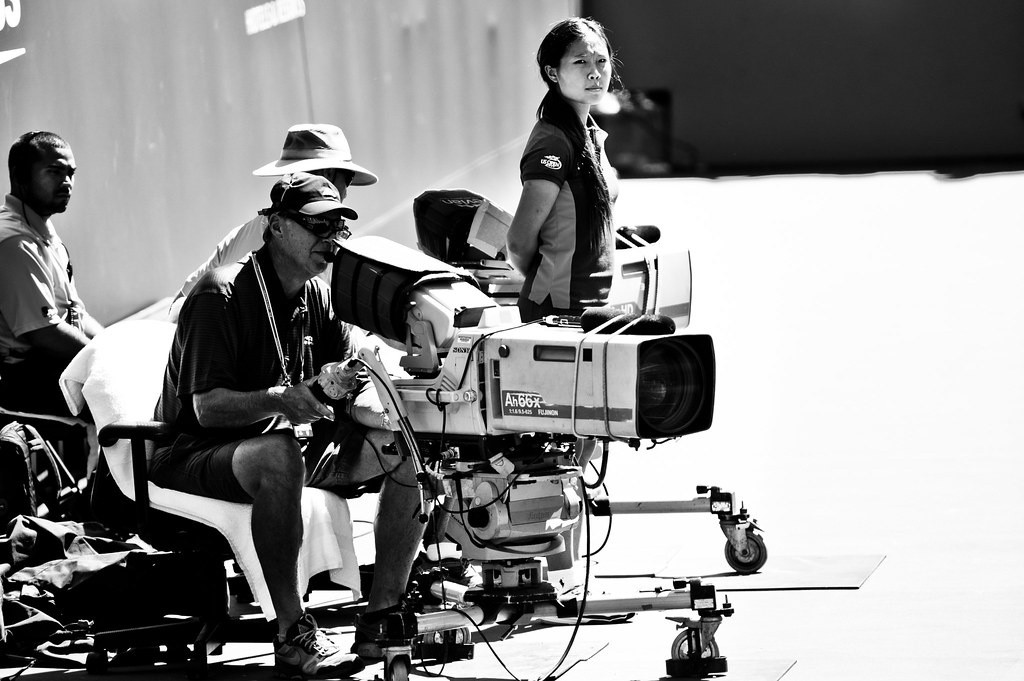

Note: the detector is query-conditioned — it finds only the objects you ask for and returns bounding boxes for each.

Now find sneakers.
[354,593,419,659]
[407,551,485,615]
[274,613,366,680]
[531,578,636,627]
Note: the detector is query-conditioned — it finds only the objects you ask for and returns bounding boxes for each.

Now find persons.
[0,130,104,427]
[156,120,416,355]
[506,13,635,626]
[143,163,451,677]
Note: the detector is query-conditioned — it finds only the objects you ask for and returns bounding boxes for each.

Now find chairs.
[86,319,359,681]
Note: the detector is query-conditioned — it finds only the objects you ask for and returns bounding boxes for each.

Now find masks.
[326,174,348,203]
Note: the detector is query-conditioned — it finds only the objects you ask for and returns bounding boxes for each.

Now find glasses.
[322,168,356,187]
[278,209,352,240]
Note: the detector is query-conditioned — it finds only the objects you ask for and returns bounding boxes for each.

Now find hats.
[252,123,379,187]
[257,172,359,221]
